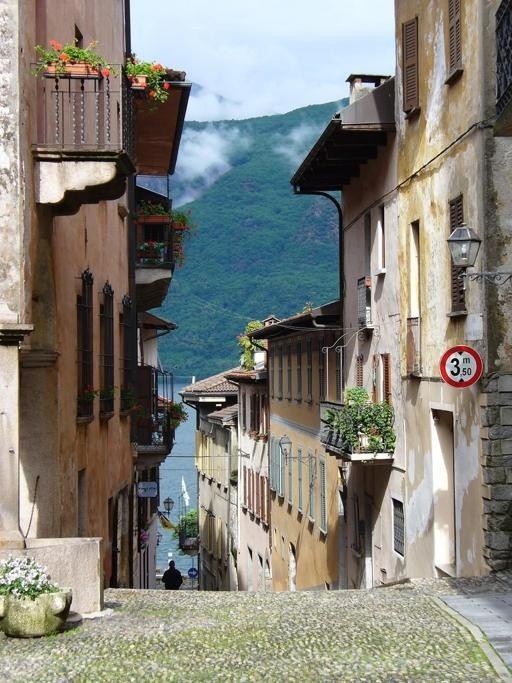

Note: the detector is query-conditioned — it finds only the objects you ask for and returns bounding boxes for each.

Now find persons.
[162,561,183,590]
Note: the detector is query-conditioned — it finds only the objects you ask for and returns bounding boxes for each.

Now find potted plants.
[317,386,397,454]
[169,508,200,552]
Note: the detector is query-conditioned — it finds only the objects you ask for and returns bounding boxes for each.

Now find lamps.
[445,223,512,292]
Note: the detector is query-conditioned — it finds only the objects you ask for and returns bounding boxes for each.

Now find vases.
[0,588,74,637]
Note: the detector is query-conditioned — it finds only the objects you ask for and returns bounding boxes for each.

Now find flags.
[182,478,190,506]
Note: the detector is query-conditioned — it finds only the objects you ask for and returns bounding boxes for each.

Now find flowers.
[28,36,172,105]
[76,381,148,421]
[0,553,59,601]
